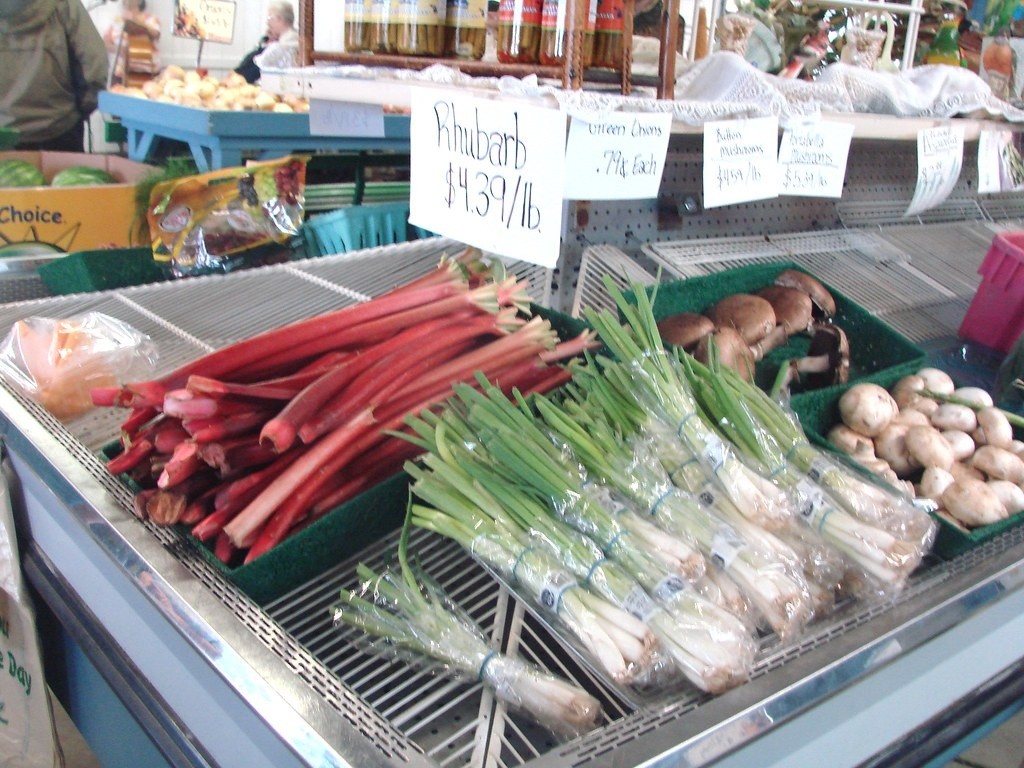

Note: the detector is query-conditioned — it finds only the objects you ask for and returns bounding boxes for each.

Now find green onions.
[329,267,936,733]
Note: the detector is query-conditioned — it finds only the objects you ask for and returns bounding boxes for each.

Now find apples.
[108,66,310,113]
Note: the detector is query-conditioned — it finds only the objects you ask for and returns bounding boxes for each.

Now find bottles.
[842,34,858,64]
[925,19,961,68]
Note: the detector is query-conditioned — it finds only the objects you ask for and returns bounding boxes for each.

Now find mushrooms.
[654,271,1024,536]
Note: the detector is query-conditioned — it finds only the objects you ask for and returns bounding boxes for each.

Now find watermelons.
[0,160,119,187]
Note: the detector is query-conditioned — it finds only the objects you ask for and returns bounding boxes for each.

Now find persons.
[631,0,687,65]
[104,0,160,155]
[0,0,110,153]
[253,0,299,68]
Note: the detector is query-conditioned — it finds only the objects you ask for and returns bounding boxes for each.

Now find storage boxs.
[616,261,926,415]
[98,296,615,604]
[0,150,168,256]
[792,372,1024,564]
